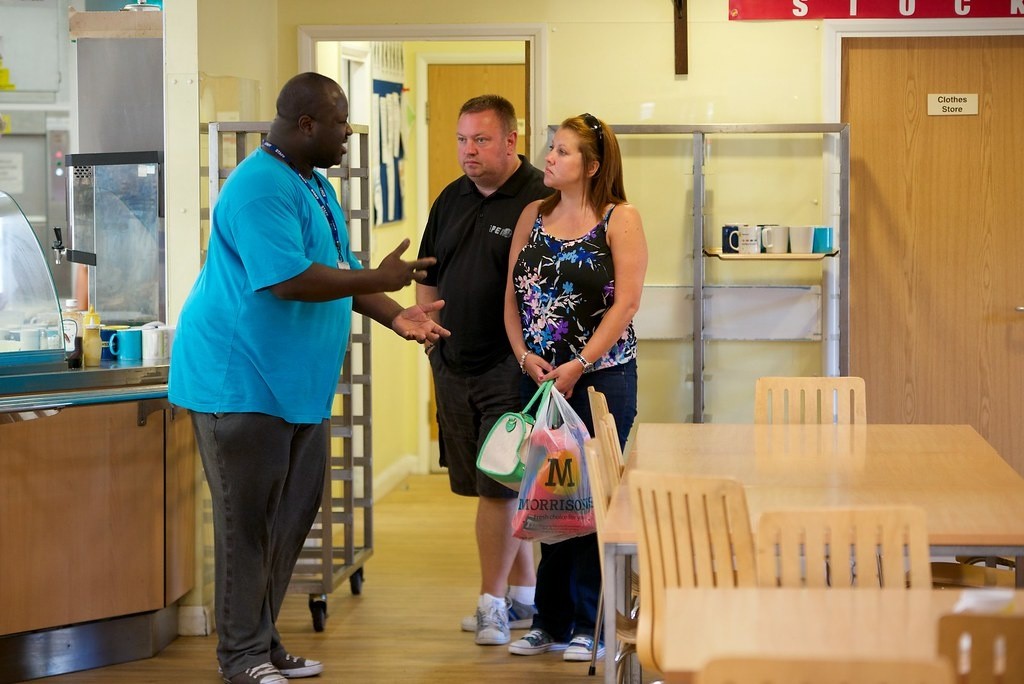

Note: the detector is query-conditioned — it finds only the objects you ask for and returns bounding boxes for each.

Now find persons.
[504,113,647,660]
[415,95,557,644]
[167,72,451,684]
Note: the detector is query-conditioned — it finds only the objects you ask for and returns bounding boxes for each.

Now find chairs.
[582,375,1024,684]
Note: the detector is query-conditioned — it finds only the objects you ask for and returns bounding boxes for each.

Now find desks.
[661,589,1024,684]
[600,424,1024,684]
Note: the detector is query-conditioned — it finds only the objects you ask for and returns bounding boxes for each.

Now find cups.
[813,227,833,253]
[722,223,788,254]
[790,226,814,254]
[100,330,117,361]
[109,330,142,363]
[102,325,130,331]
[140,325,176,360]
[19,328,39,351]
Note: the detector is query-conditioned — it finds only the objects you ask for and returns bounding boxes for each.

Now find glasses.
[579,113,605,168]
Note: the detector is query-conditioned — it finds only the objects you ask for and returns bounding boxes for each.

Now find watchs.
[575,354,594,373]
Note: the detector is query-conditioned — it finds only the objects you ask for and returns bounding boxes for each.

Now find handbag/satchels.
[476,381,558,494]
[511,385,597,545]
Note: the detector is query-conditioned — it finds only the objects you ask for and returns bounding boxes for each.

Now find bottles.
[40,331,48,350]
[83,304,101,326]
[83,317,102,367]
[61,299,82,369]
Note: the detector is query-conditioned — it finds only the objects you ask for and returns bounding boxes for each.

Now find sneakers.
[563,635,605,661]
[508,629,568,656]
[475,593,511,645]
[462,596,538,631]
[223,662,289,684]
[218,653,322,677]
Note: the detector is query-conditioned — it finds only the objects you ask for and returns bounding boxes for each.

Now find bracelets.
[519,350,533,376]
[425,343,435,354]
